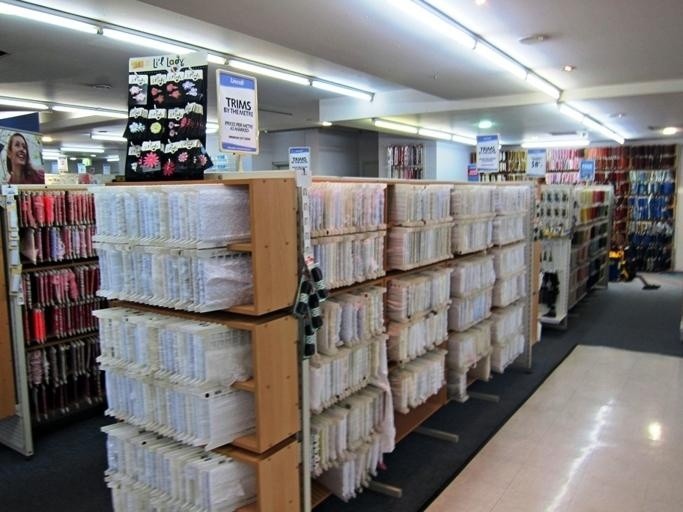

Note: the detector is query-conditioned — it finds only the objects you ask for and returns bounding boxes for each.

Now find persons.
[5,133,43,184]
